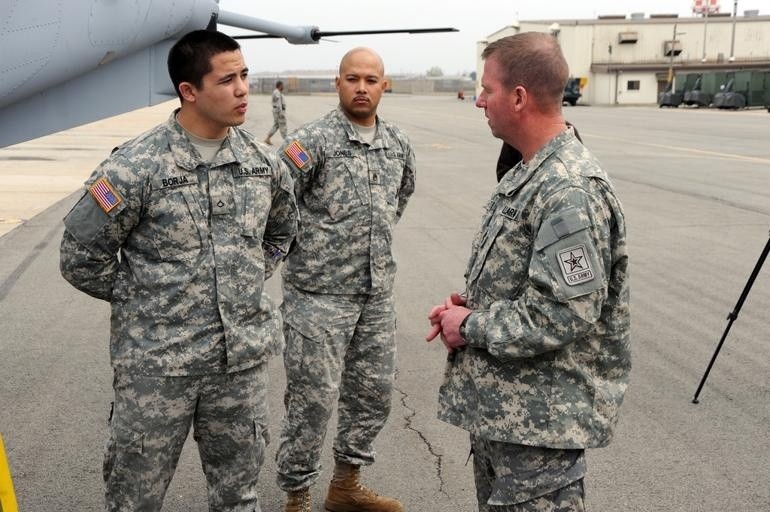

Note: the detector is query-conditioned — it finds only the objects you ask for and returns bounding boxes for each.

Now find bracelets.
[459,312,472,343]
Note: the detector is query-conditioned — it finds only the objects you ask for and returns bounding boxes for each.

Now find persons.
[264,80,289,145]
[427,31,634,511]
[59,29,302,511]
[274,47,416,511]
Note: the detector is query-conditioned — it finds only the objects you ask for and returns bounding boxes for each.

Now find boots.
[284,487,315,512]
[265,134,275,145]
[325,462,403,511]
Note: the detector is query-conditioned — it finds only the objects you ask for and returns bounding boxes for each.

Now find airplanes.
[2,1,460,149]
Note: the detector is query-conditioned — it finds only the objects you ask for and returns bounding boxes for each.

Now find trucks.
[563,77,584,107]
